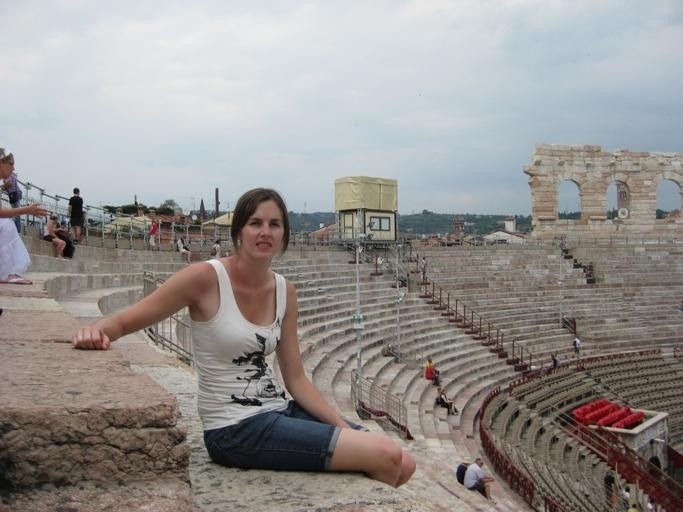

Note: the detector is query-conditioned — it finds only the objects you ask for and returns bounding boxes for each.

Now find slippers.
[0,277,31,284]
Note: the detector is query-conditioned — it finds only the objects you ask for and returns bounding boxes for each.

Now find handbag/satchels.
[456,462,470,484]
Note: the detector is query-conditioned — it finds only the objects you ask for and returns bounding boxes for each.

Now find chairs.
[570,397,648,431]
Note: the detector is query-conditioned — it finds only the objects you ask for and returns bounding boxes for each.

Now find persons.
[550,353,556,368]
[626,504,639,511]
[419,257,427,281]
[646,499,655,512]
[618,486,632,510]
[554,350,560,367]
[183,235,192,252]
[71,187,416,489]
[148,219,157,252]
[68,188,83,245]
[55,221,75,259]
[455,462,487,500]
[375,255,387,270]
[602,469,615,508]
[1,171,23,234]
[462,457,497,502]
[41,214,67,260]
[79,211,90,244]
[571,336,580,360]
[424,357,457,416]
[586,261,593,275]
[0,147,51,285]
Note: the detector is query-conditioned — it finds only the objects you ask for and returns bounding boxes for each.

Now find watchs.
[176,236,192,264]
[211,239,221,259]
